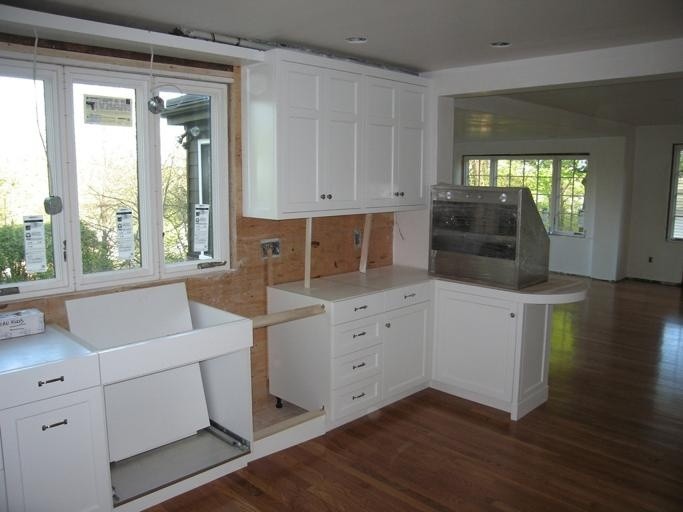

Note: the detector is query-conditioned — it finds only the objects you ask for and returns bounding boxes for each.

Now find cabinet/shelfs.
[0,353,115,512]
[241,60,368,221]
[266,281,547,422]
[368,64,430,213]
